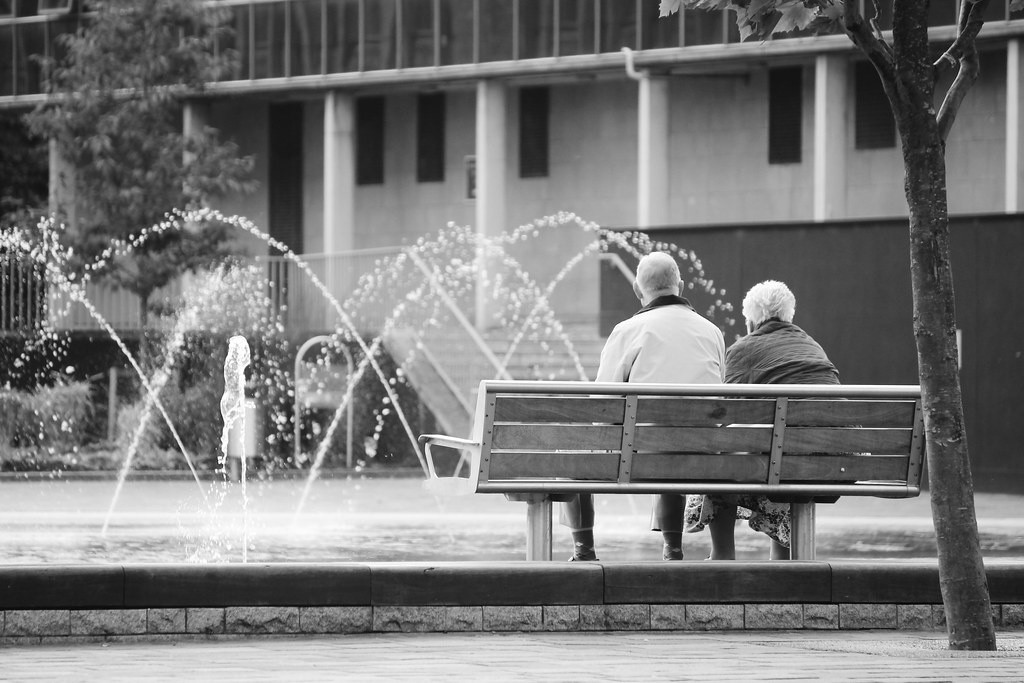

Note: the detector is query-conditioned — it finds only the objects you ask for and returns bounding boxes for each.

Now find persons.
[685,278,864,562]
[558,251,728,562]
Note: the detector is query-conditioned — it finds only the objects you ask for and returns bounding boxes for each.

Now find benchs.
[418,379,928,560]
